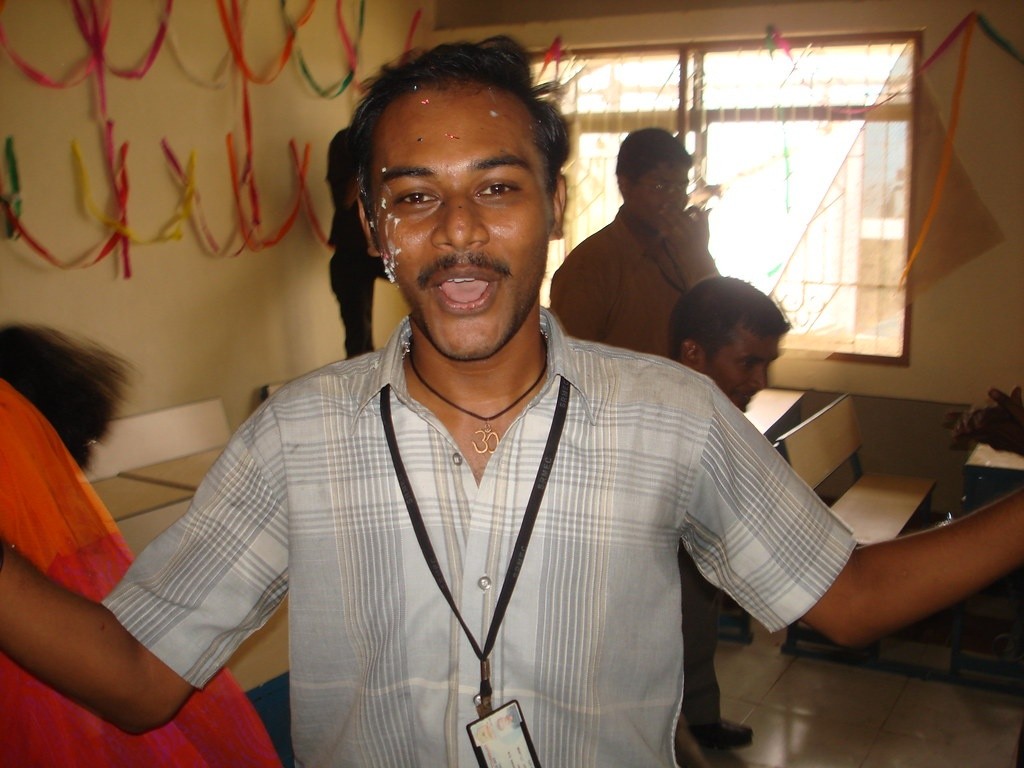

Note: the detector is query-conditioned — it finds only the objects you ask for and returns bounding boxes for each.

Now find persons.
[549,126,756,750]
[667,273,789,417]
[0,35,1024,768]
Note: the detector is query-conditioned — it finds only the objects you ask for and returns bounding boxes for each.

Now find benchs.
[84,396,231,484]
[770,391,937,669]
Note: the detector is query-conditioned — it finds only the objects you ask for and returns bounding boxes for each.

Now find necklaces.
[408,332,548,455]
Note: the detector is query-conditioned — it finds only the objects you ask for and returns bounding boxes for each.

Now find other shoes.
[690,720,754,748]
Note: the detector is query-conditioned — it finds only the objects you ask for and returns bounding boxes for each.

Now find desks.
[90,446,293,768]
[948,441,1023,684]
[713,388,806,646]
